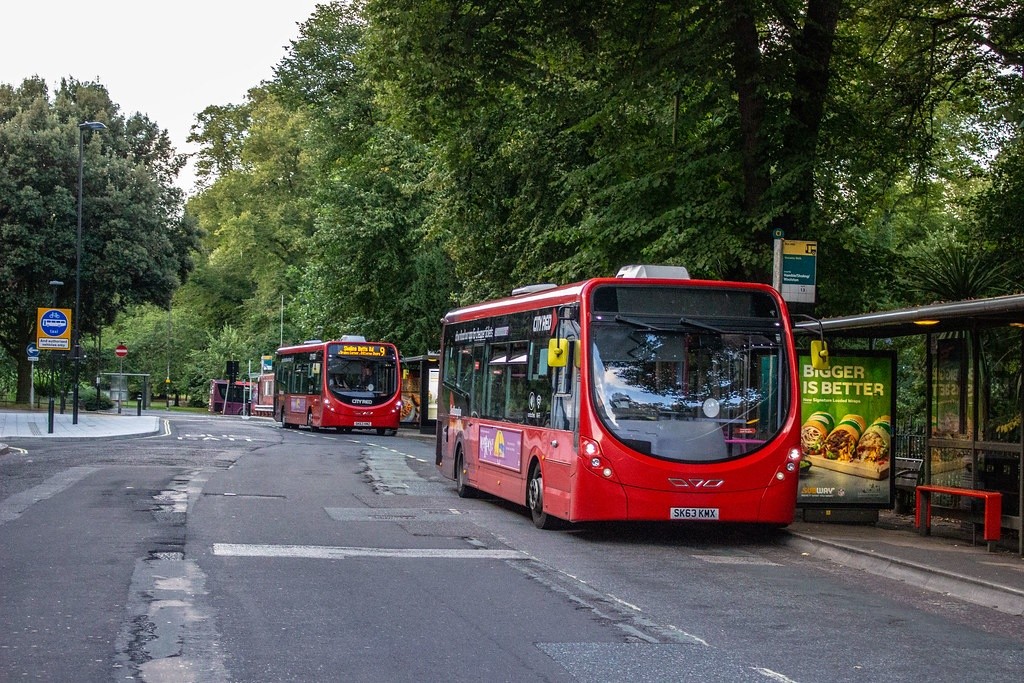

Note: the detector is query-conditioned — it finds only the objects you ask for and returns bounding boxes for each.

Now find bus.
[436,266,830,533]
[208,379,256,417]
[273,335,410,435]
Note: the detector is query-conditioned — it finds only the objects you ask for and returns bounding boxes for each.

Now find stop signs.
[116,346,128,358]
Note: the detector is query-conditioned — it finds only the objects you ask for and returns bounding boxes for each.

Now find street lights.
[72,122,108,429]
[47,282,66,434]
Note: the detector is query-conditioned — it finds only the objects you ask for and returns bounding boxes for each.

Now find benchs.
[915,484,1003,552]
